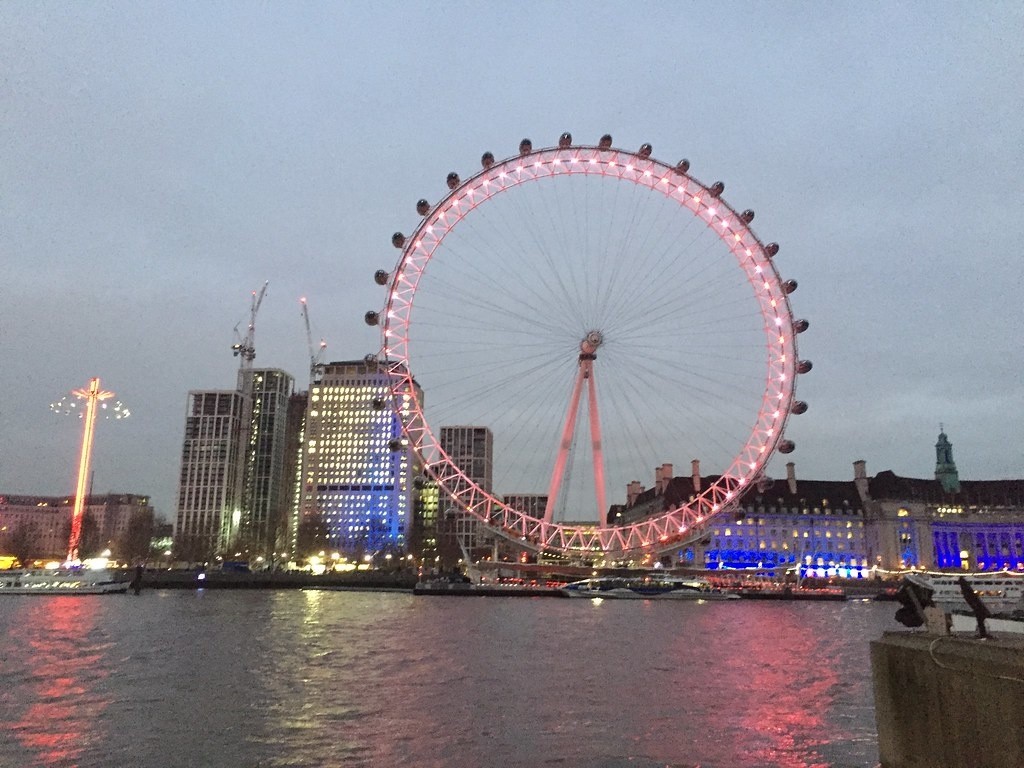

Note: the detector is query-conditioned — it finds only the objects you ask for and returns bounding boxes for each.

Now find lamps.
[894,576,943,634]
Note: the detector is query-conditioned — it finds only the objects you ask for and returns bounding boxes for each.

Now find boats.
[556,577,741,602]
[413,572,845,599]
[925,577,1024,605]
[0,560,142,595]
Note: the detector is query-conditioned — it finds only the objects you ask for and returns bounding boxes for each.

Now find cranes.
[299,297,329,385]
[229,279,271,393]
